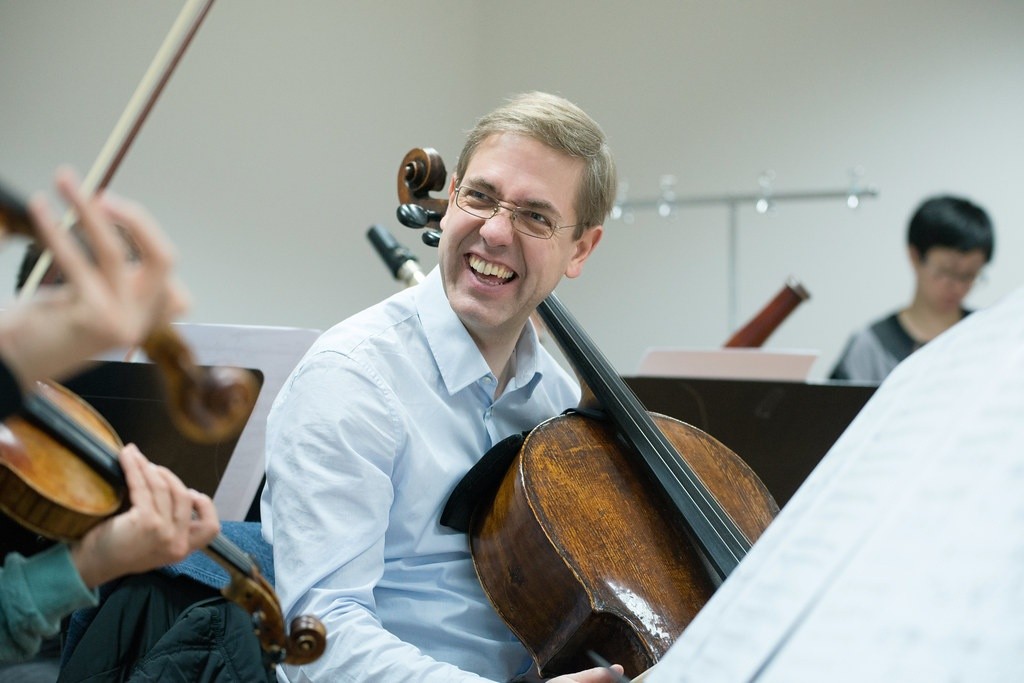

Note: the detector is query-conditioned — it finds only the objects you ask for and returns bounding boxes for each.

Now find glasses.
[454,185,592,239]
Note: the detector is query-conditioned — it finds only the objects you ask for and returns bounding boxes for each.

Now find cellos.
[397,144,782,680]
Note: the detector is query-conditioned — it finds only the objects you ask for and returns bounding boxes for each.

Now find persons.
[825,193,997,384]
[0,172,274,683]
[258,90,626,683]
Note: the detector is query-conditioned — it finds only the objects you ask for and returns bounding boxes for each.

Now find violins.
[0,377,329,669]
[1,179,263,448]
